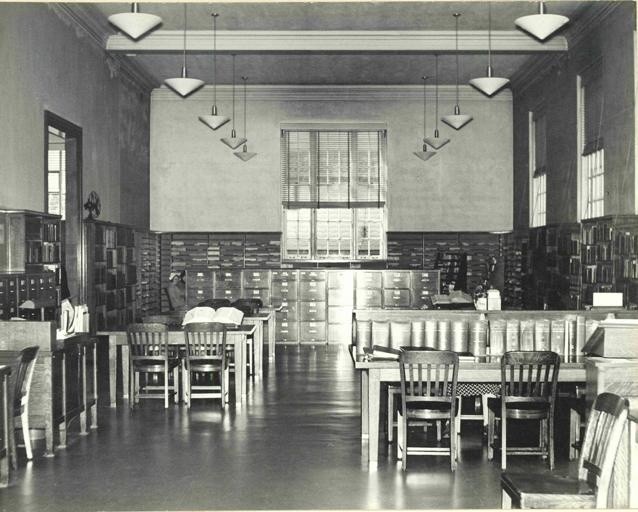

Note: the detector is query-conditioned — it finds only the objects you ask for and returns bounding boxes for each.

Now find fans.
[82,189,105,222]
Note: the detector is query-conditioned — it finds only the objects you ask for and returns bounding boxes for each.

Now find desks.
[94,320,259,413]
[351,354,589,475]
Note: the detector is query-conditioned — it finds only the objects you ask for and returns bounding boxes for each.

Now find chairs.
[181,322,234,412]
[127,320,181,413]
[9,345,42,472]
[1,362,12,483]
[501,392,630,509]
[397,350,459,473]
[487,350,561,472]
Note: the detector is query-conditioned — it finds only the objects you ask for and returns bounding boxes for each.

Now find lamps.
[414,2,571,163]
[107,3,258,164]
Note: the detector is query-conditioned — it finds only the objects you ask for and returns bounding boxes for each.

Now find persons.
[168,271,185,311]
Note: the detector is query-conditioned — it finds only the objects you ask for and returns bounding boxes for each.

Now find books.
[180,306,245,327]
[362,342,478,362]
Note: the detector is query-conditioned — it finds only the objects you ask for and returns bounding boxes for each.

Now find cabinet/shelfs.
[160,230,530,347]
[529,215,638,311]
[82,220,160,331]
[1,209,69,322]
